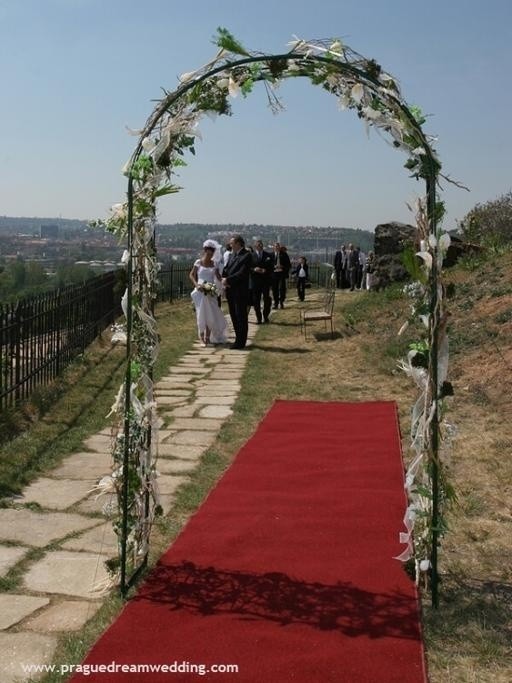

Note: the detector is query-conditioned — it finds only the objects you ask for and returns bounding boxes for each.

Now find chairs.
[299,271,335,339]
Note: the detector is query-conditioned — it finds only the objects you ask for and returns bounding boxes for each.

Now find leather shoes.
[200,336,206,348]
[263,319,270,324]
[256,320,262,325]
[337,285,364,292]
[297,296,305,301]
[272,304,278,309]
[229,342,245,350]
[280,304,285,309]
[206,336,213,347]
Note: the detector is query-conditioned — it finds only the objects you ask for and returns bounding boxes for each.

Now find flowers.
[200,281,216,295]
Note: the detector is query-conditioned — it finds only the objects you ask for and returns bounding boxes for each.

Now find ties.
[258,251,262,260]
[228,252,237,270]
[274,252,279,265]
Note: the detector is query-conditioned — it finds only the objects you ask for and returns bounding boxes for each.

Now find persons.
[188,234,376,349]
